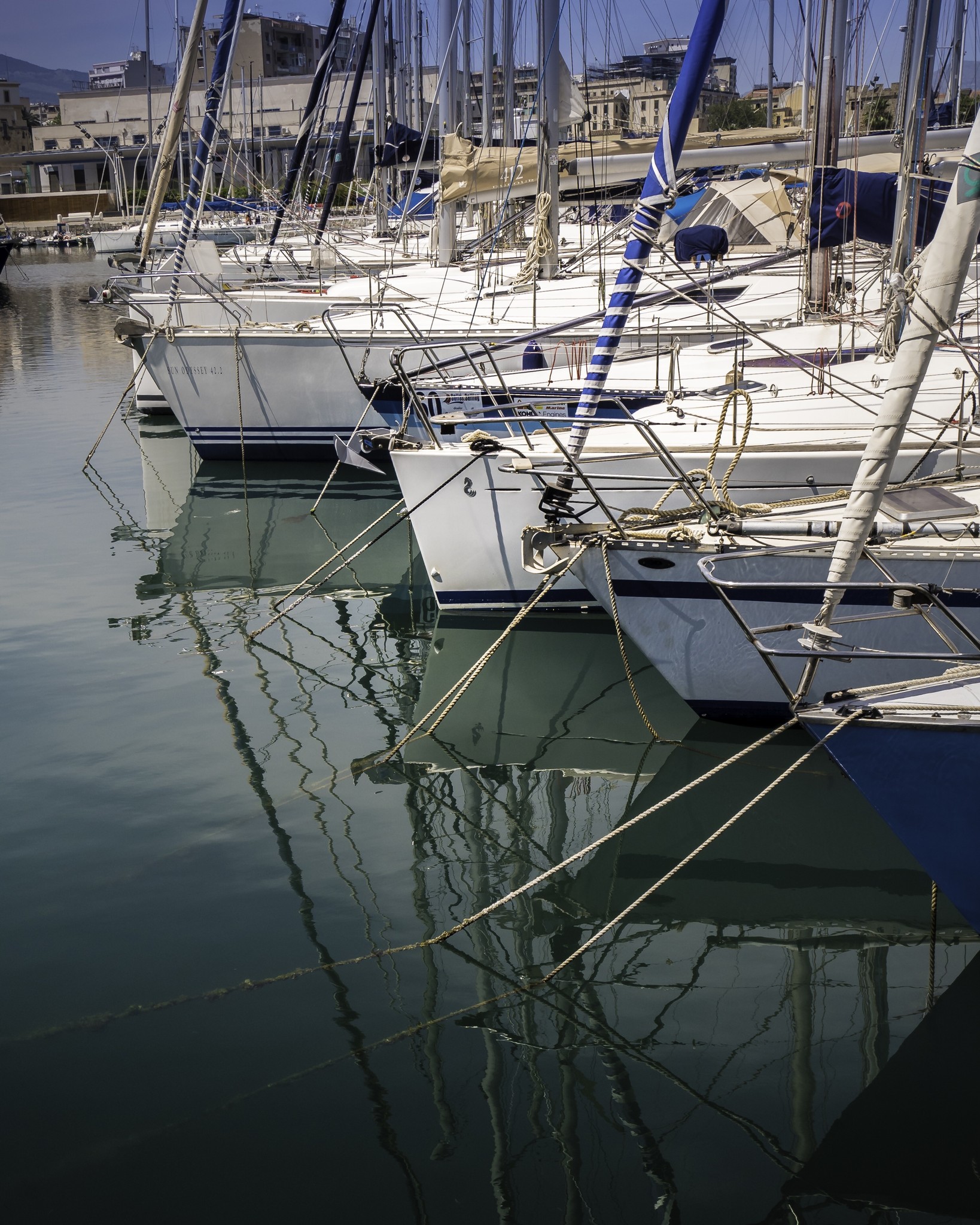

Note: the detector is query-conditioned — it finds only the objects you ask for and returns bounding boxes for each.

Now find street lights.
[75,123,125,222]
[132,114,168,219]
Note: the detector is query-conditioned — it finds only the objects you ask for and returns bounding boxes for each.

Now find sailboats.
[0,0,980,942]
